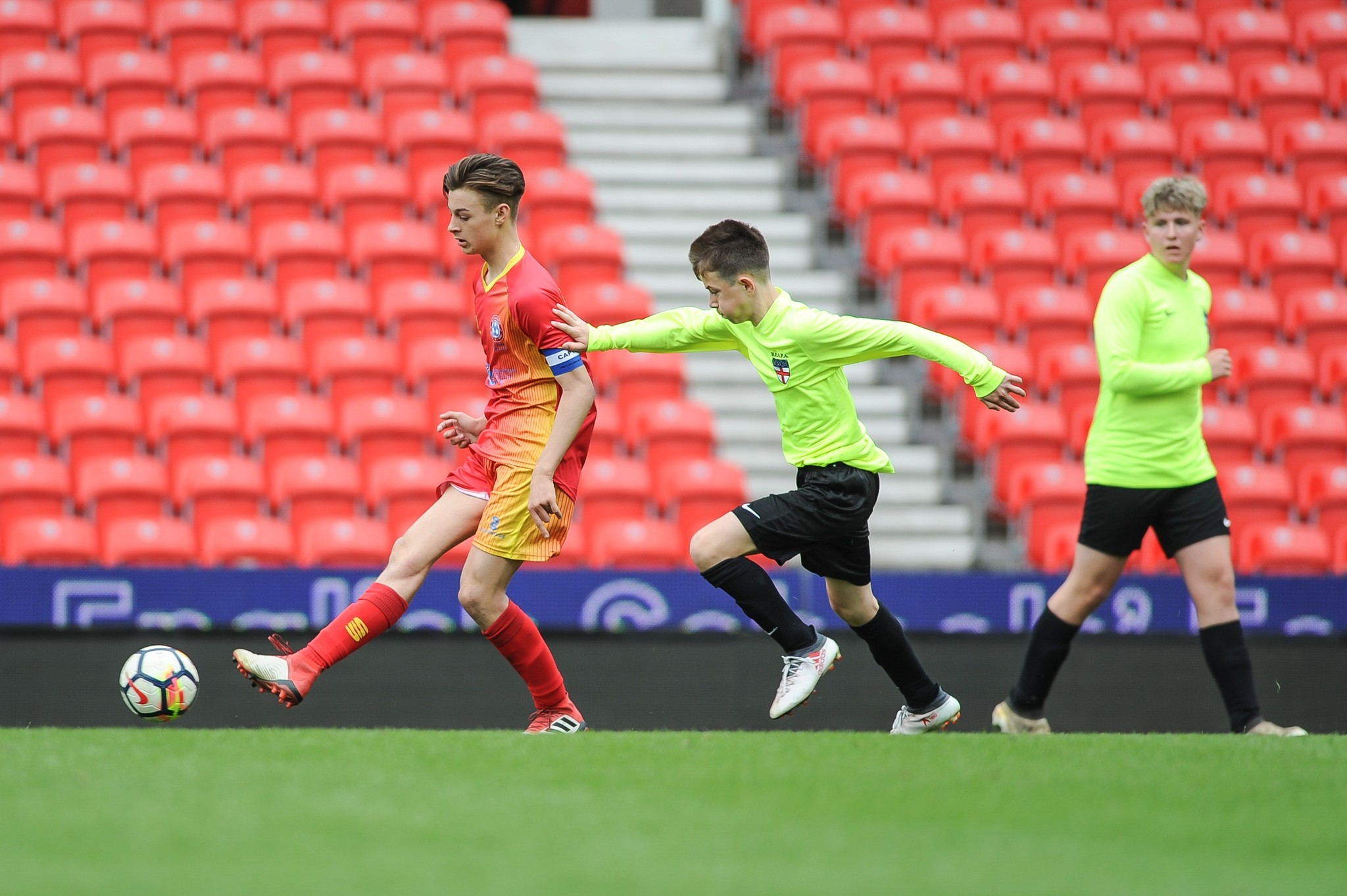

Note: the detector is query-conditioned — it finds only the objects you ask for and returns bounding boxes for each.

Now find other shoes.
[990,702,1051,735]
[1242,717,1308,738]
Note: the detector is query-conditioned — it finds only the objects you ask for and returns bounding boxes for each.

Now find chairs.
[0,0,1347,576]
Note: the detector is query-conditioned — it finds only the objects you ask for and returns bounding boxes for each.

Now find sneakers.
[524,694,589,735]
[233,633,323,708]
[891,681,961,736]
[769,624,843,720]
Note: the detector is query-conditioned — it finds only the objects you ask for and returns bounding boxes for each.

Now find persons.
[990,174,1308,736]
[232,153,596,734]
[551,219,1026,733]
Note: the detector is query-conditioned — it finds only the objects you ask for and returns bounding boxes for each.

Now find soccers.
[117,644,200,724]
